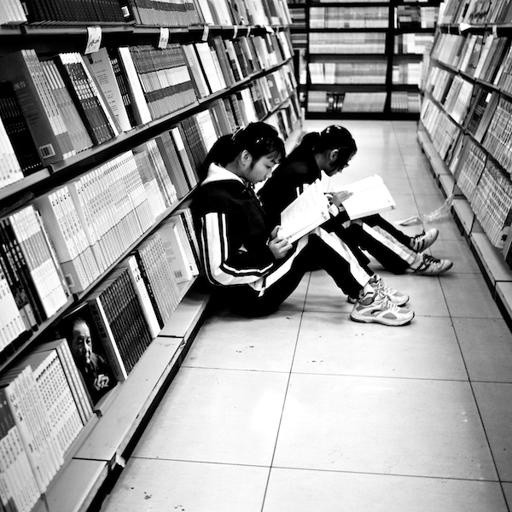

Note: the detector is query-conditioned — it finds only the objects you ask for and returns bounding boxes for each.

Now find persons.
[188,119,417,326]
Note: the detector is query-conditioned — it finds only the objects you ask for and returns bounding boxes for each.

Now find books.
[288,5,440,115]
[420,2,509,259]
[1,0,297,186]
[1,96,304,512]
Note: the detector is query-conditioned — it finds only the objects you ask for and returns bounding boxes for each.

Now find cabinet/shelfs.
[287,0,445,120]
[0,0,305,510]
[417,0,512,319]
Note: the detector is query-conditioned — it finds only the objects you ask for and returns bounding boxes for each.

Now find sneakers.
[411,228,439,253]
[348,273,415,326]
[405,252,453,276]
